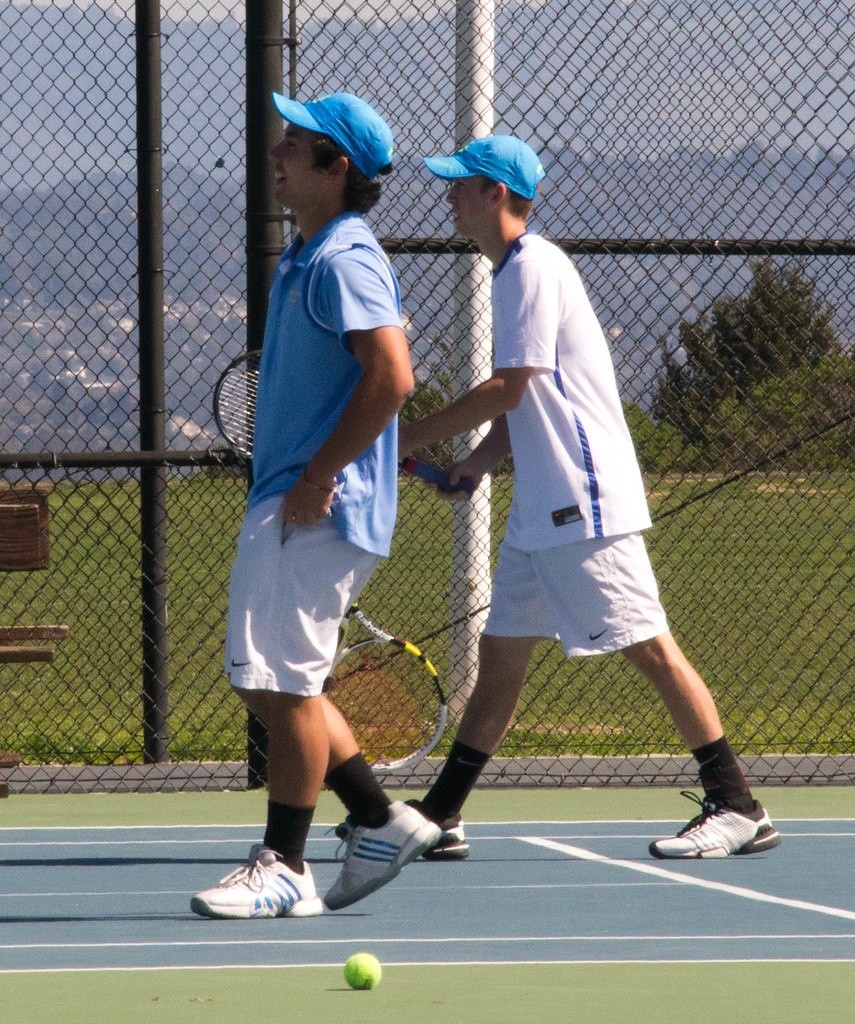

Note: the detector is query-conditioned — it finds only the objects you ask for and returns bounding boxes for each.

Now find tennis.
[342,954,382,991]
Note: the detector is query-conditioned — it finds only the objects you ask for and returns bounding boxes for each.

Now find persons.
[189,90,445,918]
[396,133,780,862]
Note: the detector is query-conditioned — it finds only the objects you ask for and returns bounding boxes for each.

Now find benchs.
[0,485,72,665]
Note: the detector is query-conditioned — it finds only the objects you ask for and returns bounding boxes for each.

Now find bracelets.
[304,466,334,492]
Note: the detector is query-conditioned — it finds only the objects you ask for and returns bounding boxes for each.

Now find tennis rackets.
[215,355,472,773]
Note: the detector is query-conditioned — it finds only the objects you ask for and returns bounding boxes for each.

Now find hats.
[424,135,546,200]
[271,92,394,179]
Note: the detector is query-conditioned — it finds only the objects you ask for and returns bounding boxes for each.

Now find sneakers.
[324,800,442,912]
[190,844,324,919]
[650,791,781,860]
[333,799,469,859]
